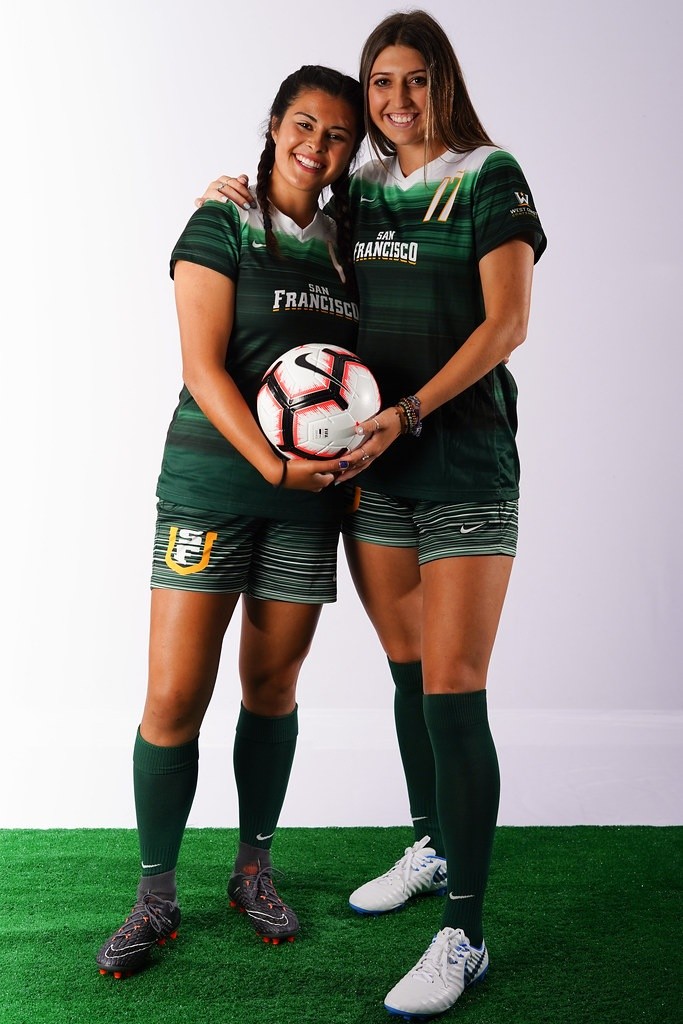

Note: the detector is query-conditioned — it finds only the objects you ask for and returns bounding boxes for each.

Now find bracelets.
[272,459,287,491]
[394,395,423,437]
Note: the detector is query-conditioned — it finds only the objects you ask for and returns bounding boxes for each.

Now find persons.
[195,7,547,1012]
[98,65,362,979]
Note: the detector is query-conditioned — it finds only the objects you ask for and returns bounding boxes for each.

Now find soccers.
[254,345,385,462]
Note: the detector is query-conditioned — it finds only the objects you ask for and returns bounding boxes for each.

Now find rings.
[226,178,232,186]
[360,447,370,461]
[372,417,380,431]
[216,182,226,191]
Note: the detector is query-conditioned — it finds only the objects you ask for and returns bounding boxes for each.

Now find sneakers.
[383,926,488,1020]
[226,857,298,944]
[97,888,181,978]
[348,834,448,914]
